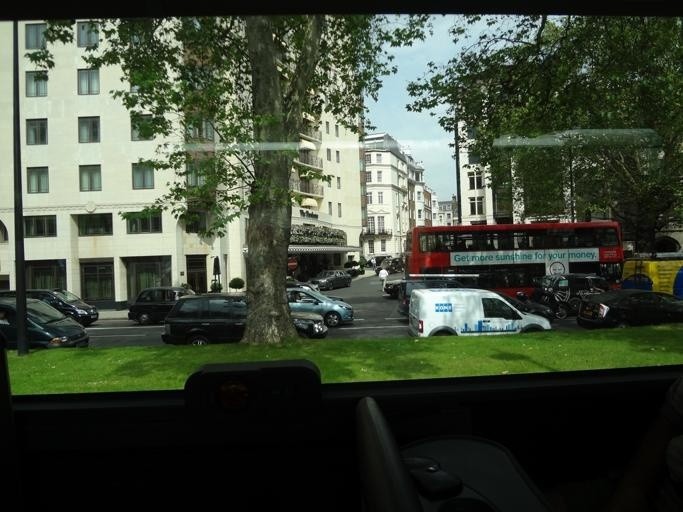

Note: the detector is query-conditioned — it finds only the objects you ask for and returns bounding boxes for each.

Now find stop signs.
[287,259,298,270]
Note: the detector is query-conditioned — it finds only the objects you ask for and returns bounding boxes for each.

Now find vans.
[406,286,552,339]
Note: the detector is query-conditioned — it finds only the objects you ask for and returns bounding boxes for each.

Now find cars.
[128,280,354,347]
[309,269,354,292]
[0,286,99,350]
[499,274,683,330]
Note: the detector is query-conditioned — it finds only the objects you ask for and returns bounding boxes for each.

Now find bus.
[403,221,626,299]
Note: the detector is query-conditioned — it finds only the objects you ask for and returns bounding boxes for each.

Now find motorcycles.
[514,288,568,320]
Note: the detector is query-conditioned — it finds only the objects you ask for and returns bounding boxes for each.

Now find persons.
[377,266,388,292]
[370,256,376,271]
